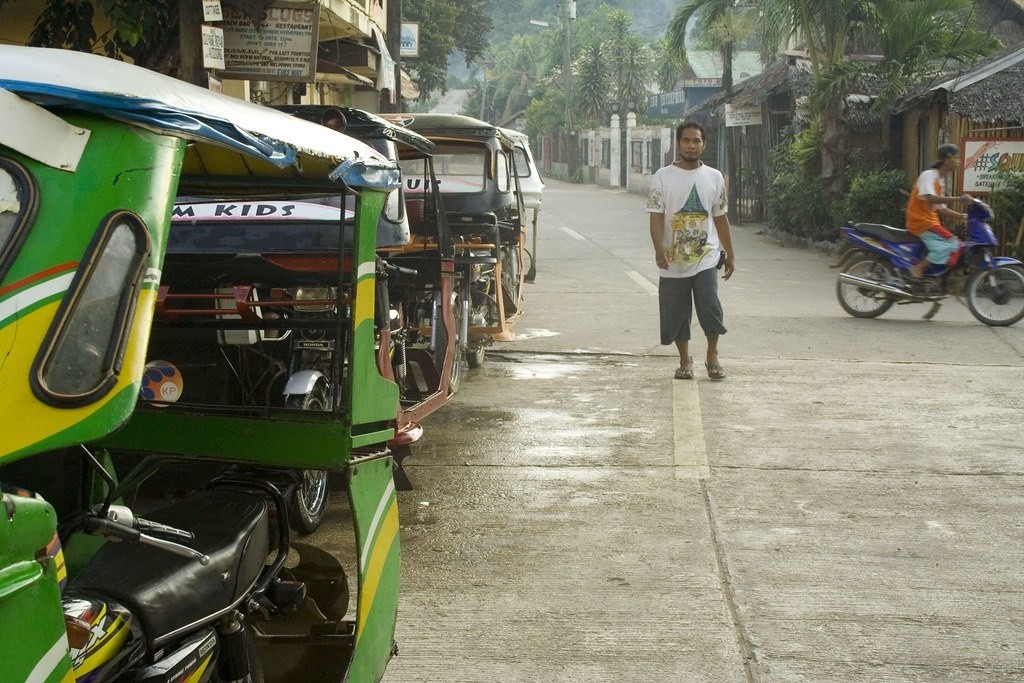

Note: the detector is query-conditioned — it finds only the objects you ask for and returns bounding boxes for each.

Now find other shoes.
[904,271,933,285]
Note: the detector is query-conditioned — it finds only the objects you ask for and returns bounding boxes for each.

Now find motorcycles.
[828,193,1024,326]
[0,44,406,683]
[184,103,546,535]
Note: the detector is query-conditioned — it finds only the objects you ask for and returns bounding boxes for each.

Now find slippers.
[705,361,725,378]
[675,368,692,379]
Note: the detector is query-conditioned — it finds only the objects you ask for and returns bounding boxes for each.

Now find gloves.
[960,193,973,205]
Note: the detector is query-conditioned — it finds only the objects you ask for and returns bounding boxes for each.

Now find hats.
[930,143,959,167]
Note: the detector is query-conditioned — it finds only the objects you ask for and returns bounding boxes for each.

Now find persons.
[902,142,975,284]
[646,121,735,379]
